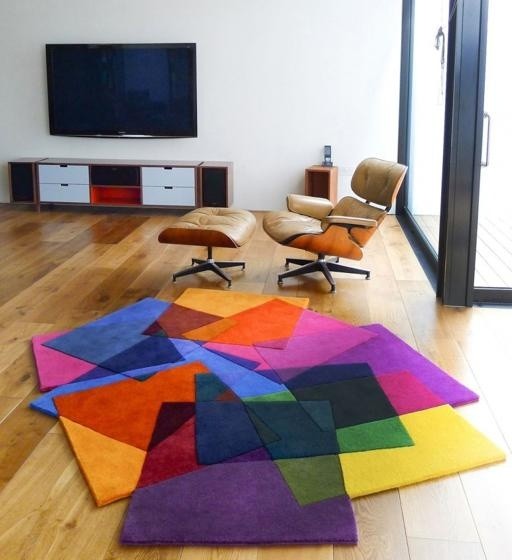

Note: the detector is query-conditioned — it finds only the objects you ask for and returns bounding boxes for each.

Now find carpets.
[28,286,506,547]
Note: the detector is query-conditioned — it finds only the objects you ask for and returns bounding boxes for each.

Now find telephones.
[323,146,332,166]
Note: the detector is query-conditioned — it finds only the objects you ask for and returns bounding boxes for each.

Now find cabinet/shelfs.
[304,164,337,202]
[6,160,235,214]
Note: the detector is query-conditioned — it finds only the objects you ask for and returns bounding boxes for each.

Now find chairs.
[262,156,409,294]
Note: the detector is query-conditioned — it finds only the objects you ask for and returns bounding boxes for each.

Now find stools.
[158,206,257,288]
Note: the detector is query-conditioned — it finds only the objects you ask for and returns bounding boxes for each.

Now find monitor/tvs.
[46,43,197,138]
